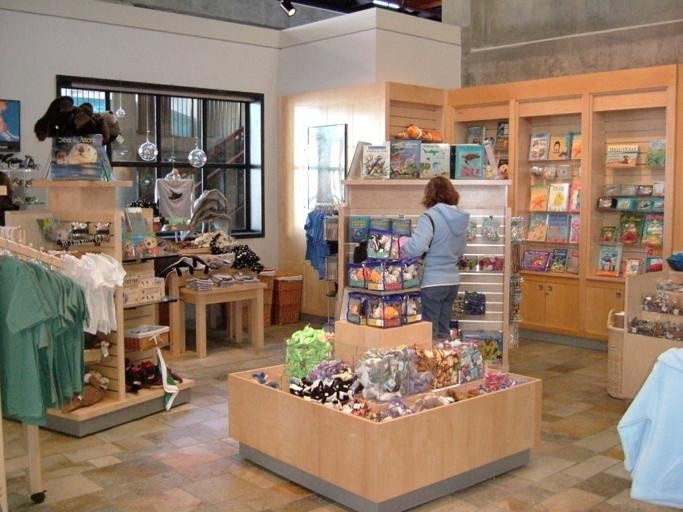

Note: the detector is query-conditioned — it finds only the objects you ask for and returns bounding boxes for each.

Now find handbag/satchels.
[463,291,486,315]
[417,259,424,280]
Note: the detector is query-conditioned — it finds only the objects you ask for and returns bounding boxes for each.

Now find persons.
[396,176,472,342]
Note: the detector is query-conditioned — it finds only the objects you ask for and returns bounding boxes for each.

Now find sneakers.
[126,361,182,390]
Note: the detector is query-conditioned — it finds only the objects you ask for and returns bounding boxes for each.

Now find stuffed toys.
[123,356,184,397]
[33,95,123,147]
[61,371,110,414]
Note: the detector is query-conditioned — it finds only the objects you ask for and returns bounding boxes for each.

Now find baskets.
[125,326,169,351]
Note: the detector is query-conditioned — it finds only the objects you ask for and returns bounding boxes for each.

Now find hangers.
[315,200,335,213]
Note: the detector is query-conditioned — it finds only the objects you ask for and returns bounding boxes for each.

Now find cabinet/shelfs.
[226,321,542,512]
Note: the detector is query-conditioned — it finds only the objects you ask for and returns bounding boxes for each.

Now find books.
[463,120,666,281]
[344,212,424,330]
[358,140,487,182]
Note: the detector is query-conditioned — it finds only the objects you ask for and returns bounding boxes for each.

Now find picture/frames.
[308,124,346,214]
[0,99,21,152]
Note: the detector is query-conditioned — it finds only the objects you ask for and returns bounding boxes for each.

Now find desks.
[181,282,267,358]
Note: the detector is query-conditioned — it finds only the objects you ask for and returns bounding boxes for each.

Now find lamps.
[280,0,296,17]
